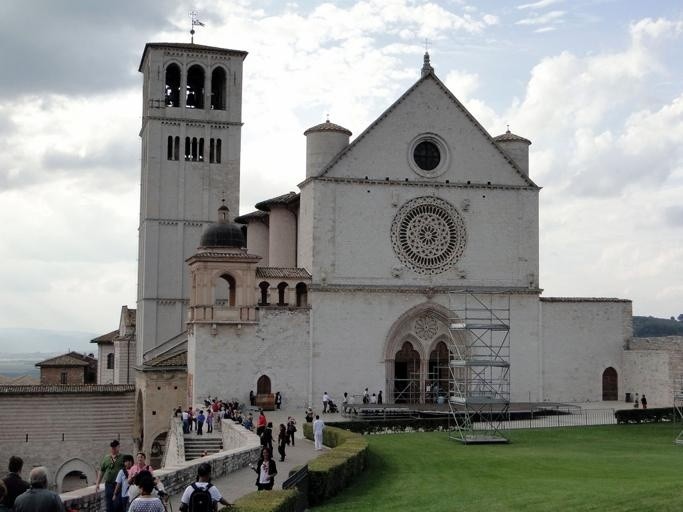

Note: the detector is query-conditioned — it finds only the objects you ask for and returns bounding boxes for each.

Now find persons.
[95,439,168,512]
[3,456,30,511]
[179,462,232,511]
[634,392,641,407]
[171,388,384,492]
[642,393,647,408]
[0,479,8,511]
[13,467,65,512]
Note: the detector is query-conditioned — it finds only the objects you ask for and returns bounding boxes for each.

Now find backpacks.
[190,483,213,512]
